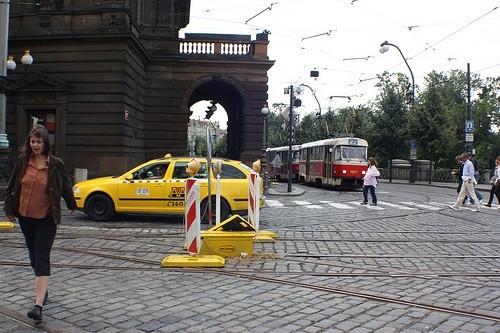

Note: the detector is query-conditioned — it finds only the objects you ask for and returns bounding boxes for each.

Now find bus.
[266,145,301,183]
[299,138,368,191]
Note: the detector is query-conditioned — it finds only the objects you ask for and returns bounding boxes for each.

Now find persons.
[3,124,78,319]
[485,155,500,206]
[451,155,469,206]
[448,151,481,212]
[147,164,167,179]
[464,152,483,205]
[360,157,380,206]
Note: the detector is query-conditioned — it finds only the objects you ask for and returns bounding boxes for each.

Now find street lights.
[379,41,415,184]
[283,85,302,194]
[260,102,269,195]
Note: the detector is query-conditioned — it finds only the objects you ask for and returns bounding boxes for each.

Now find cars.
[72,158,266,224]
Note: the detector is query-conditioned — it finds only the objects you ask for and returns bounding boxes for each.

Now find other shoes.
[467,202,473,206]
[35,290,49,306]
[484,204,491,207]
[369,202,377,206]
[471,208,480,212]
[360,202,368,205]
[448,205,458,212]
[27,305,42,326]
[478,199,484,204]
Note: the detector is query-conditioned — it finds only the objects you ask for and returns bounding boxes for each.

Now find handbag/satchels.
[6,191,19,211]
